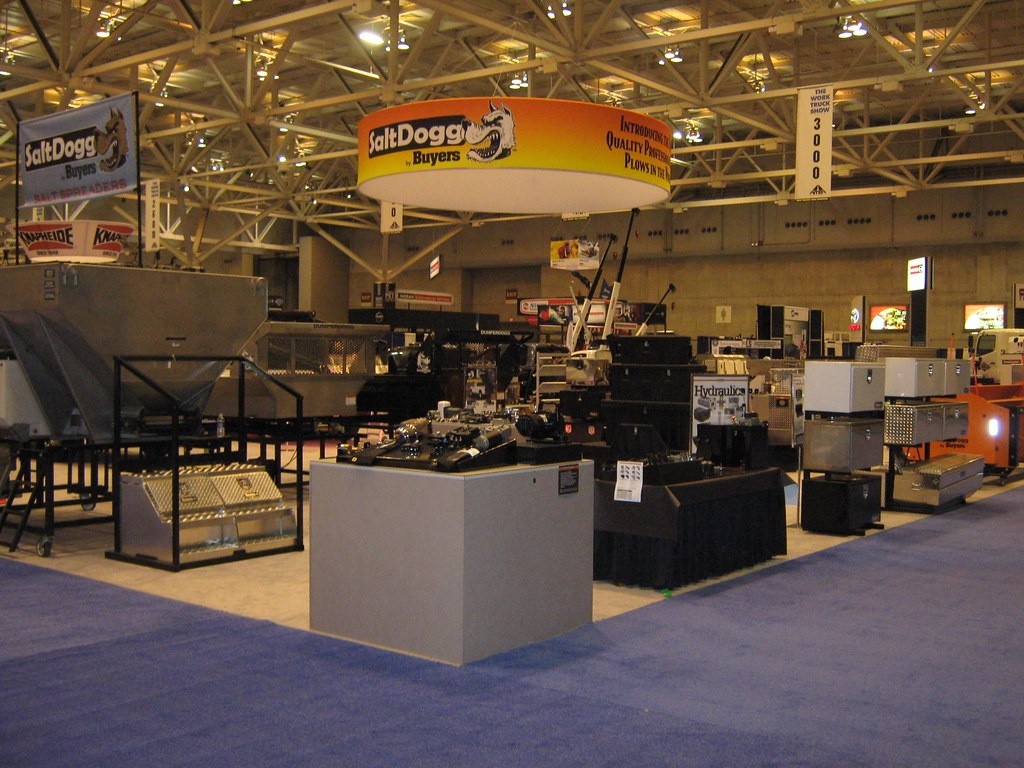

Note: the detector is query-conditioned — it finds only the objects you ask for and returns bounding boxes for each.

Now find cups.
[438,401,450,418]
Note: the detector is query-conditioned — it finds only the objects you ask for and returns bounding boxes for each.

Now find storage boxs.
[802,356,986,535]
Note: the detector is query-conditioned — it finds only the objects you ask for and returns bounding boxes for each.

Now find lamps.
[0,0,986,204]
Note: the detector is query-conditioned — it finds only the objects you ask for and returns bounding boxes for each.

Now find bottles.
[507,377,520,405]
[217,413,225,437]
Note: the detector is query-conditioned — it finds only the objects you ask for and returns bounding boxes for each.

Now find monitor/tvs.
[961,301,1007,333]
[869,303,910,333]
